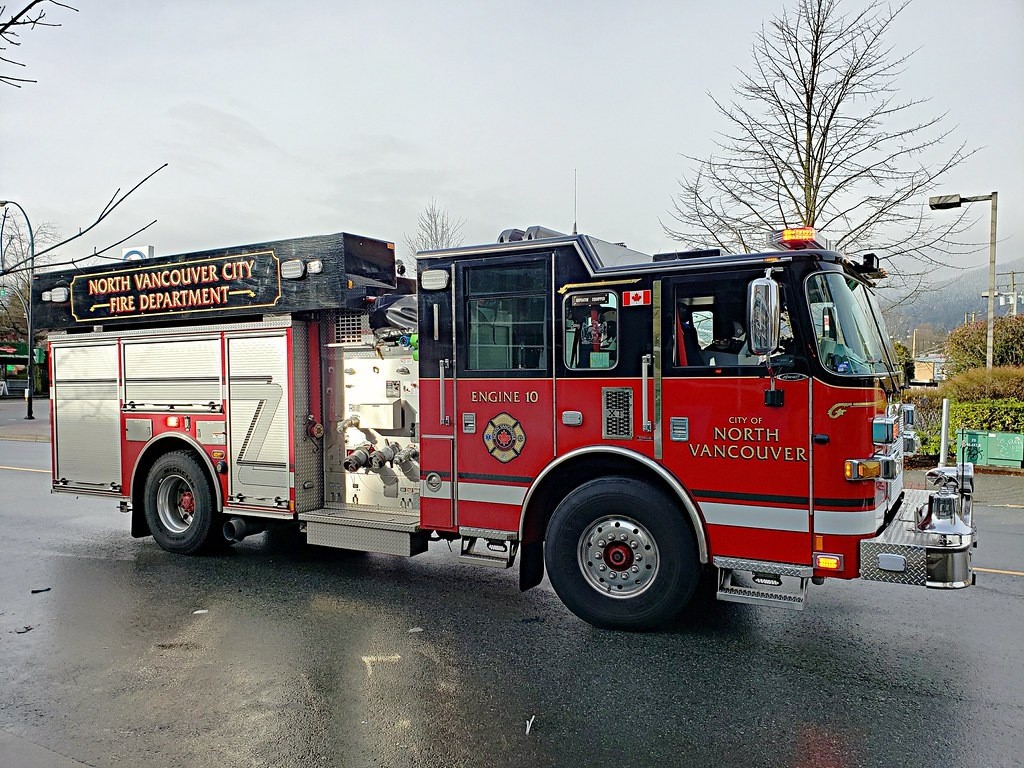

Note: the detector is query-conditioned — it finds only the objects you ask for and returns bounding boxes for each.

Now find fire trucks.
[32,226,977,631]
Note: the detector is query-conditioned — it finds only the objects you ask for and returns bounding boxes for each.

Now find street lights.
[929,194,997,366]
[982,291,1018,314]
[0,201,35,419]
